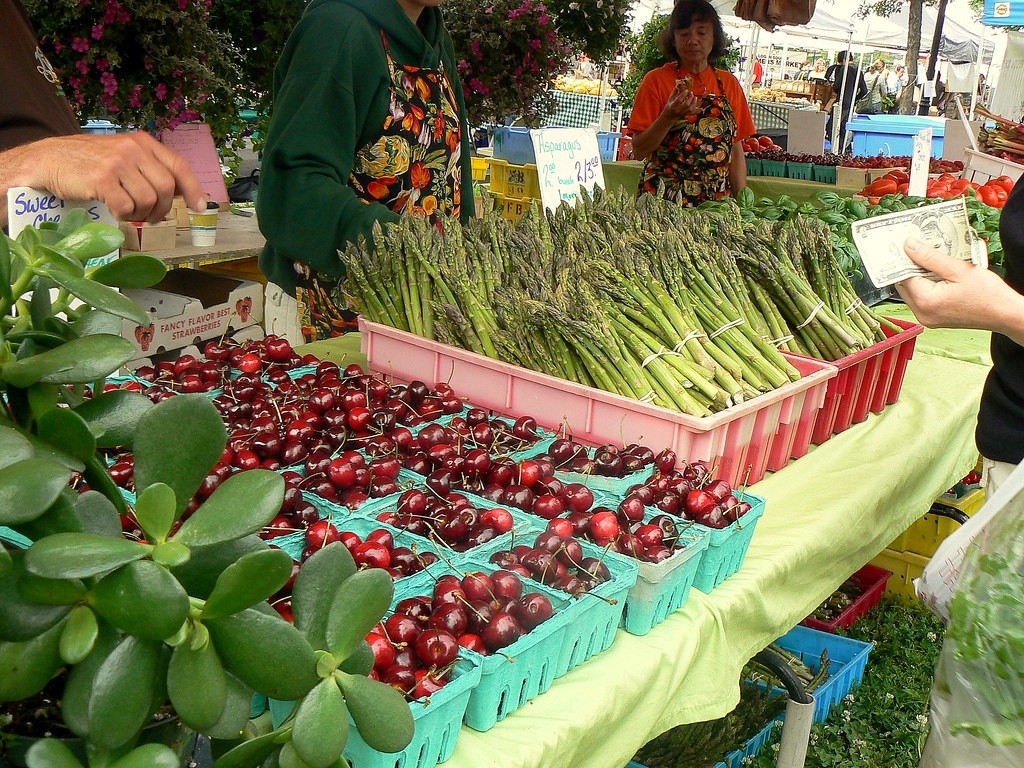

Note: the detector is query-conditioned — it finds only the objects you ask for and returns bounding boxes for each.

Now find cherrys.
[746,149,850,166]
[52,311,750,707]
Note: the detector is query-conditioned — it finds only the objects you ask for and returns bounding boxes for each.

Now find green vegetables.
[943,515,1023,750]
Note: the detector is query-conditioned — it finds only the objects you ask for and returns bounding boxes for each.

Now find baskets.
[0,349,765,768]
[742,159,837,185]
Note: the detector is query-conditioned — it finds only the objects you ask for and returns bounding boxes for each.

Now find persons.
[255,0,478,311]
[794,58,991,121]
[749,54,763,90]
[824,51,869,155]
[892,168,1024,505]
[626,0,758,209]
[1,0,209,238]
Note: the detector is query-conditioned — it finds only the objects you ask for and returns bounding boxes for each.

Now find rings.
[897,281,904,287]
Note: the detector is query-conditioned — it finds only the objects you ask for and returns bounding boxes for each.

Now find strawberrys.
[841,155,965,173]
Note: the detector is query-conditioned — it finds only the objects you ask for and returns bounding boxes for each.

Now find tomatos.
[859,170,1015,209]
[740,135,783,153]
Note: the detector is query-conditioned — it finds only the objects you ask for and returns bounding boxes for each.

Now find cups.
[186,202,220,247]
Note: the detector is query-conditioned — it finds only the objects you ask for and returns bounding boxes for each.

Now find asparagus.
[631,644,835,768]
[337,187,906,421]
[810,574,863,622]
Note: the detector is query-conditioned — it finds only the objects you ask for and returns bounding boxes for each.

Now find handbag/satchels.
[855,92,874,114]
[911,456,1024,621]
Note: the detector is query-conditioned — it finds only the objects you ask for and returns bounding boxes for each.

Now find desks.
[601,158,866,215]
[534,91,634,132]
[269,331,993,767]
[114,204,281,300]
[748,98,814,128]
[869,302,998,365]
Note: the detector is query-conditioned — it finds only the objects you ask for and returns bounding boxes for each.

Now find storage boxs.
[92,76,1024,768]
